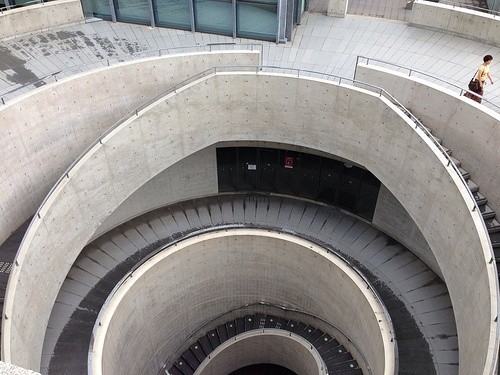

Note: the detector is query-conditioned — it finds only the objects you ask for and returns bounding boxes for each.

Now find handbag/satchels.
[468,78,482,94]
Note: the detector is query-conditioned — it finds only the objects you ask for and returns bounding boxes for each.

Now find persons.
[463,54,493,104]
